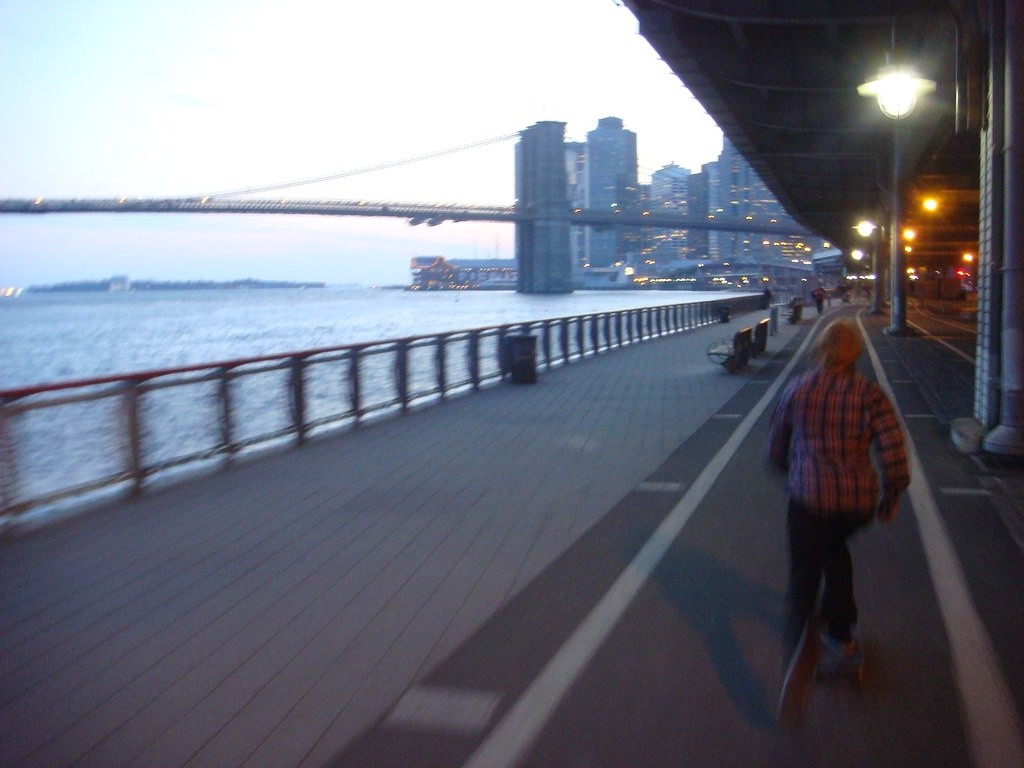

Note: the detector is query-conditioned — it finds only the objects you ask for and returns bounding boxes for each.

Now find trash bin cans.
[719,306,731,322]
[505,333,538,385]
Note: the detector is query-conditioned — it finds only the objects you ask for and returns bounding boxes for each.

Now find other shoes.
[822,630,857,658]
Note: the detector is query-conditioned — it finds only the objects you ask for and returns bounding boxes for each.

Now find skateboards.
[780,612,821,726]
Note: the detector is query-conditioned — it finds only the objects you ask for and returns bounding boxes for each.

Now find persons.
[812,283,827,314]
[767,316,910,689]
[763,286,771,310]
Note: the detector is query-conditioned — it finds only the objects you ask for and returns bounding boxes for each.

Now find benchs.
[709,303,803,374]
